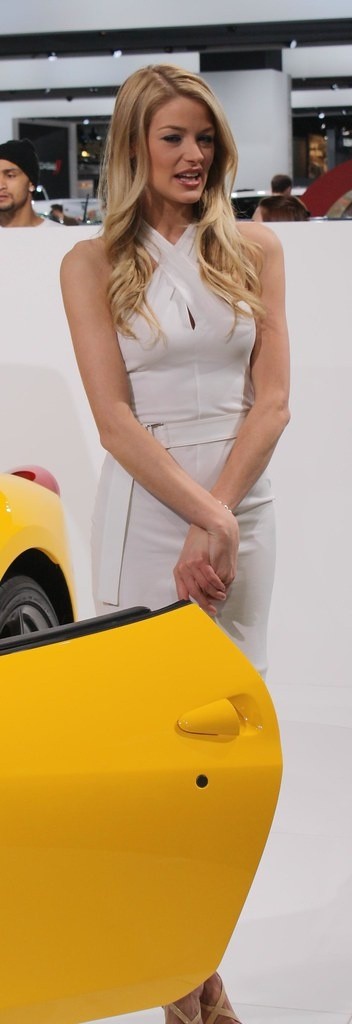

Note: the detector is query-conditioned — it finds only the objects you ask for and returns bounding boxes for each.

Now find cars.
[229,183,309,221]
[0,460,286,1024]
[31,195,102,225]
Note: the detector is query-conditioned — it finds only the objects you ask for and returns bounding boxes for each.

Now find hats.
[0,137,40,186]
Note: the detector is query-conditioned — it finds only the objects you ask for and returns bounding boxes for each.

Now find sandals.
[199,971,243,1024]
[162,993,202,1024]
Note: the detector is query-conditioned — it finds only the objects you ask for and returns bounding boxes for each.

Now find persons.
[0,139,63,228]
[251,175,310,222]
[51,204,79,226]
[61,64,291,1024]
